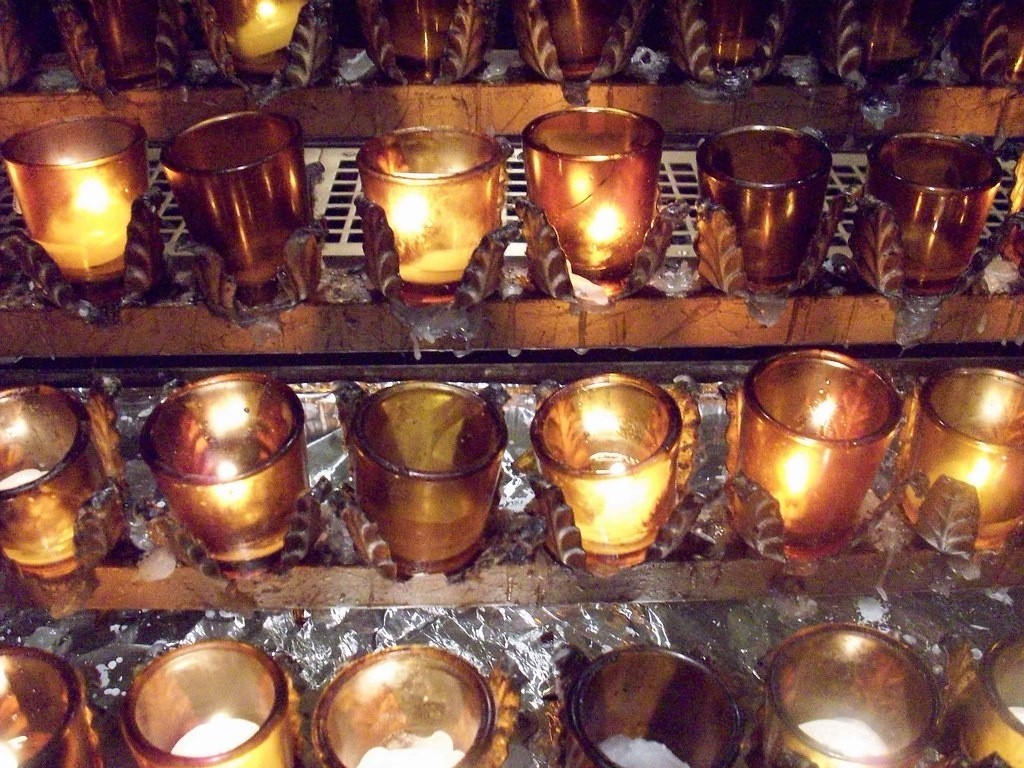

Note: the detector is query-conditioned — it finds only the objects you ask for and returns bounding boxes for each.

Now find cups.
[0,0,1024,768]
